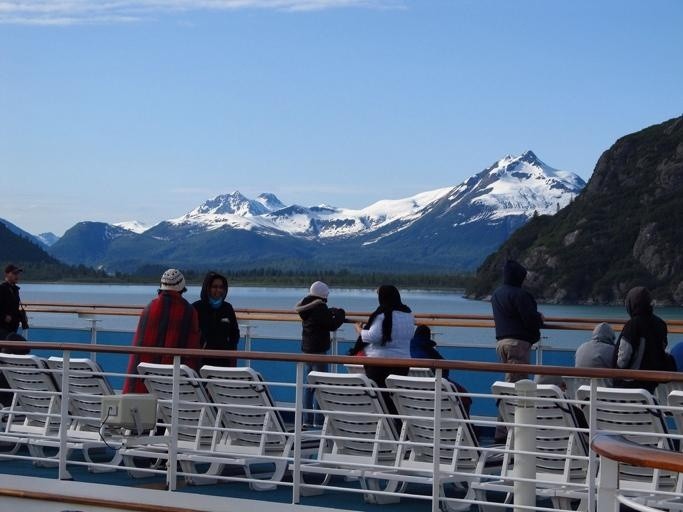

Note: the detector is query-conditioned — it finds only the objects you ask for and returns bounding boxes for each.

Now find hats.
[308,280,330,299]
[3,264,23,275]
[157,268,185,292]
[0,332,30,354]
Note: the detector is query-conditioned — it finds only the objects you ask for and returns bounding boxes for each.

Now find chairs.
[574,386,673,511]
[308,368,413,507]
[197,364,324,496]
[0,353,75,473]
[667,390,683,512]
[47,355,140,476]
[384,374,512,512]
[488,381,588,512]
[126,363,221,484]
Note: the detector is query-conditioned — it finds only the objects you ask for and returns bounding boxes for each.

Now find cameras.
[20,310,29,329]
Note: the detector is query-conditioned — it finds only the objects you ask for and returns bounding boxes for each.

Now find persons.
[533,372,589,436]
[0,332,59,411]
[0,265,29,342]
[294,280,346,428]
[573,322,618,389]
[490,261,548,442]
[612,286,668,394]
[409,323,473,418]
[354,284,414,436]
[189,271,240,401]
[121,268,200,465]
